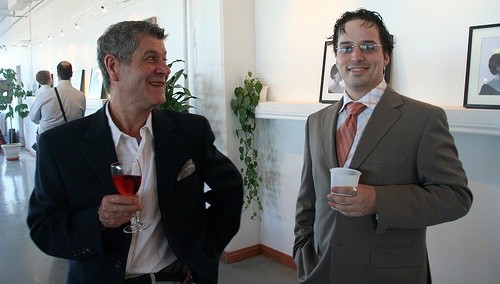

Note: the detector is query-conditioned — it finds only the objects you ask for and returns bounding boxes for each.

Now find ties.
[335,102,367,169]
[335,45,383,54]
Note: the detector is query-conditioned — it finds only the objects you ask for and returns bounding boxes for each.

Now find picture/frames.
[87,68,105,99]
[71,69,84,91]
[318,34,394,102]
[463,23,500,108]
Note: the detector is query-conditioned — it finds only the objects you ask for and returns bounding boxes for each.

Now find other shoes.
[32,143,37,150]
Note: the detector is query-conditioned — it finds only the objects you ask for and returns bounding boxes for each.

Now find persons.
[29,60,86,136]
[27,21,243,284]
[294,8,473,284]
[31,70,53,151]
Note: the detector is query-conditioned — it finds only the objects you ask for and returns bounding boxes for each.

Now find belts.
[125,272,187,284]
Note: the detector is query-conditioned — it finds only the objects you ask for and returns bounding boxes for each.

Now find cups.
[330,167,361,210]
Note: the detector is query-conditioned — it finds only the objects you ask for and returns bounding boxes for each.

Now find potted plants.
[0,68,38,161]
[231,72,269,220]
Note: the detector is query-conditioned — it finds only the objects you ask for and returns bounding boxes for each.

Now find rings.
[352,187,357,197]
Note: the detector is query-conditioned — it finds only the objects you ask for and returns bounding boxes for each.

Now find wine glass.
[111,157,152,233]
